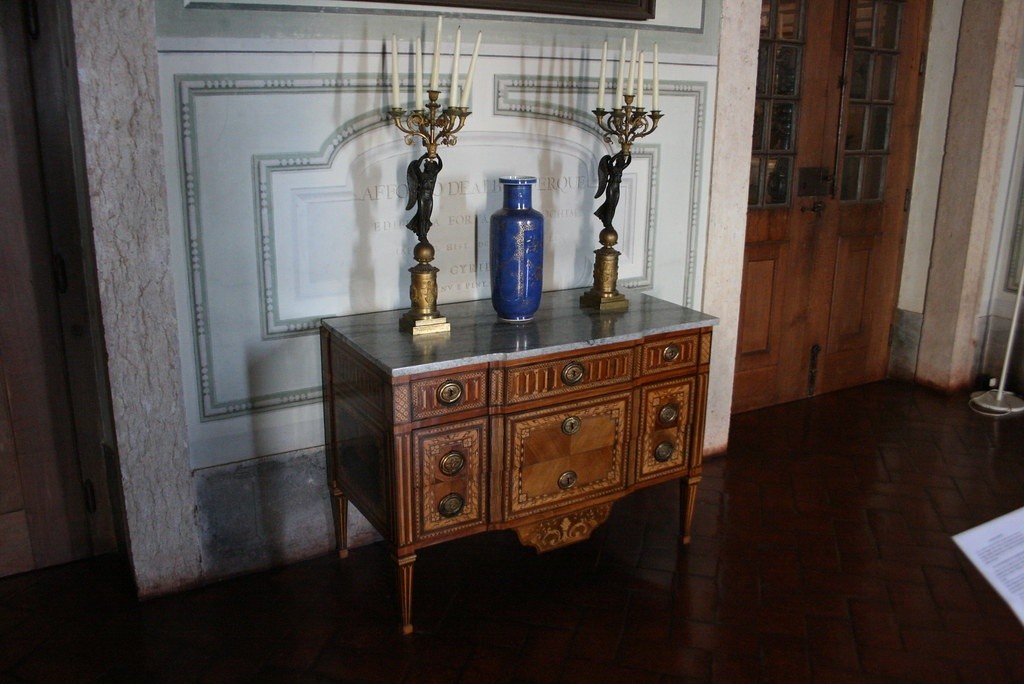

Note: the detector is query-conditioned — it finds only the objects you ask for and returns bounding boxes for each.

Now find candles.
[392,35,401,107]
[651,41,660,110]
[460,30,482,107]
[449,26,461,107]
[637,50,645,109]
[416,36,424,110]
[626,28,638,95]
[597,39,608,107]
[614,36,627,109]
[430,14,443,90]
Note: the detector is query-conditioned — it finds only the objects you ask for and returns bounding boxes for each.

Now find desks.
[318,286,720,635]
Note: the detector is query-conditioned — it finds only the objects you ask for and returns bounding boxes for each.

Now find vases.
[488,177,543,322]
